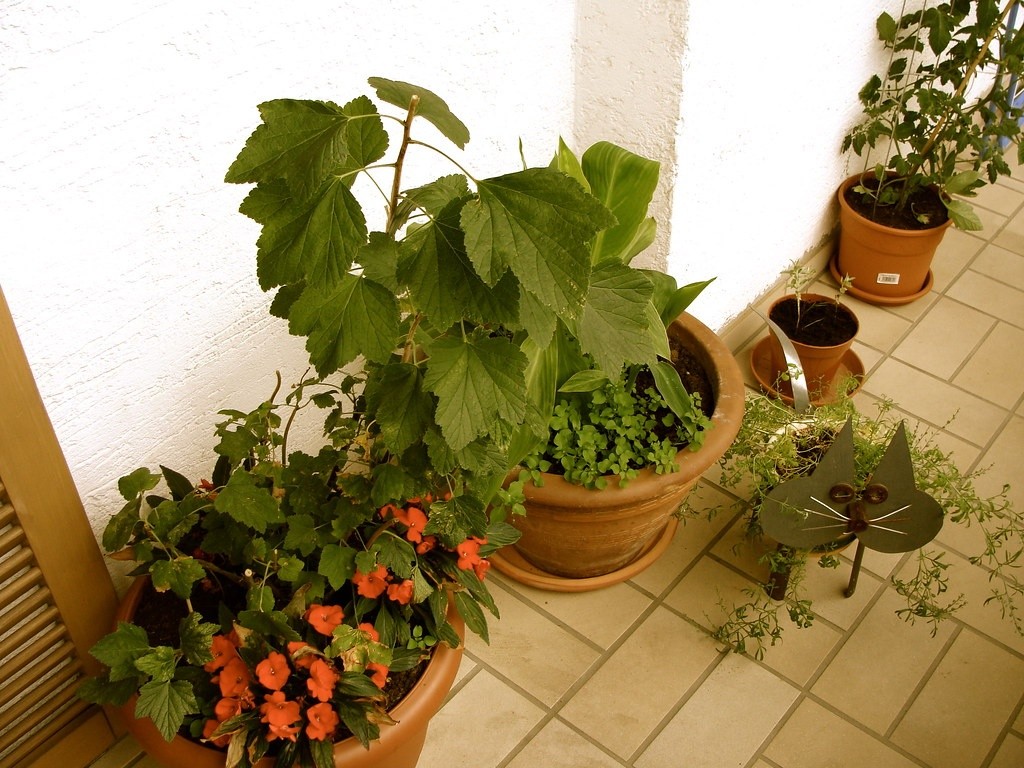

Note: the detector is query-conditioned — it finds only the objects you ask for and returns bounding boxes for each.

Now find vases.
[105,523,466,766]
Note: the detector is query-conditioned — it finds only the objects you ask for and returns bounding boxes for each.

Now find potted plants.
[224,74,746,595]
[715,362,1023,661]
[831,0,1024,304]
[751,260,866,410]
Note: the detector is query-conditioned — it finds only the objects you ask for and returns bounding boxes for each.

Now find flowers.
[67,369,521,767]
